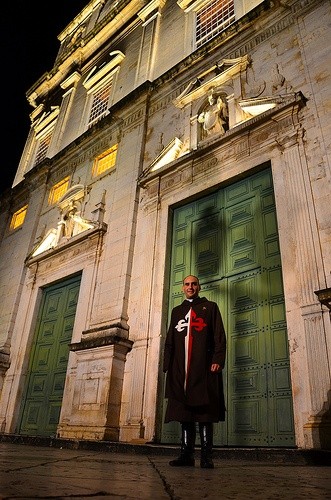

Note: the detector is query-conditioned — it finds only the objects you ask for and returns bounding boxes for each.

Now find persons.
[162,274,228,469]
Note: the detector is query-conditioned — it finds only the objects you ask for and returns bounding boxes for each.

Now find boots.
[169,425,196,466]
[199,424,214,469]
